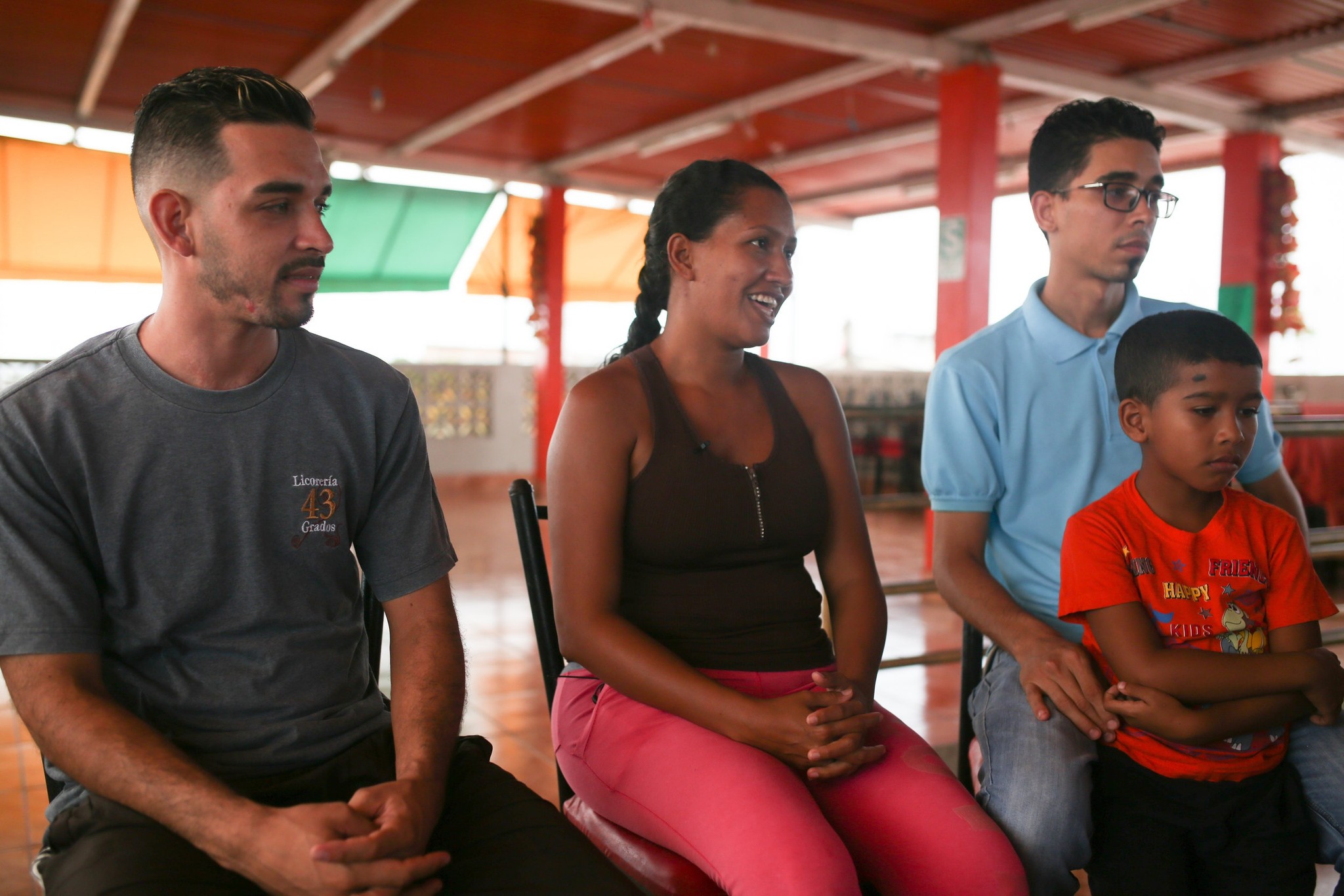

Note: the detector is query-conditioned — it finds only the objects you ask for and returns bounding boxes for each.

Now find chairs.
[509,478,732,896]
[959,622,992,794]
[361,571,383,690]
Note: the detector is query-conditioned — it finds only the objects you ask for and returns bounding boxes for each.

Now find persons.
[540,158,1037,896]
[919,96,1344,896]
[0,65,642,896]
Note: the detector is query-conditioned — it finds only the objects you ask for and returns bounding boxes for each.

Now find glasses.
[1049,181,1178,222]
